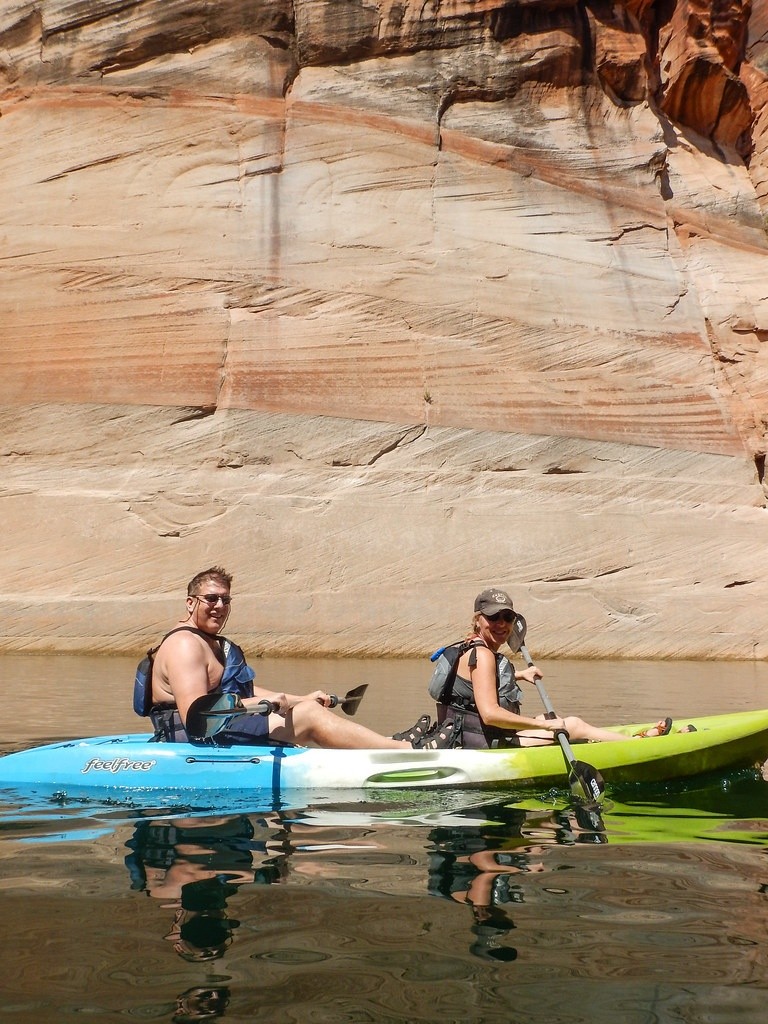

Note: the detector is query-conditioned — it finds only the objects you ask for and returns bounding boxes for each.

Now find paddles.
[506,613,606,810]
[186,684,369,735]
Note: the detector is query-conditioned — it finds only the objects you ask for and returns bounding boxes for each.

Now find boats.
[1,708,768,812]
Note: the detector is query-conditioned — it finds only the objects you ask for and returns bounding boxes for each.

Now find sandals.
[632,718,673,739]
[392,714,432,741]
[676,724,696,734]
[411,717,455,750]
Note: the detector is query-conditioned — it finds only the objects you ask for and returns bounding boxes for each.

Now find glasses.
[480,612,515,623]
[188,594,233,605]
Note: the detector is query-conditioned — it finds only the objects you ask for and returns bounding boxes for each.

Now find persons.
[428,588,697,747]
[133,566,459,751]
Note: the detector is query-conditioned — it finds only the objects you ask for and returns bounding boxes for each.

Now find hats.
[474,588,516,616]
